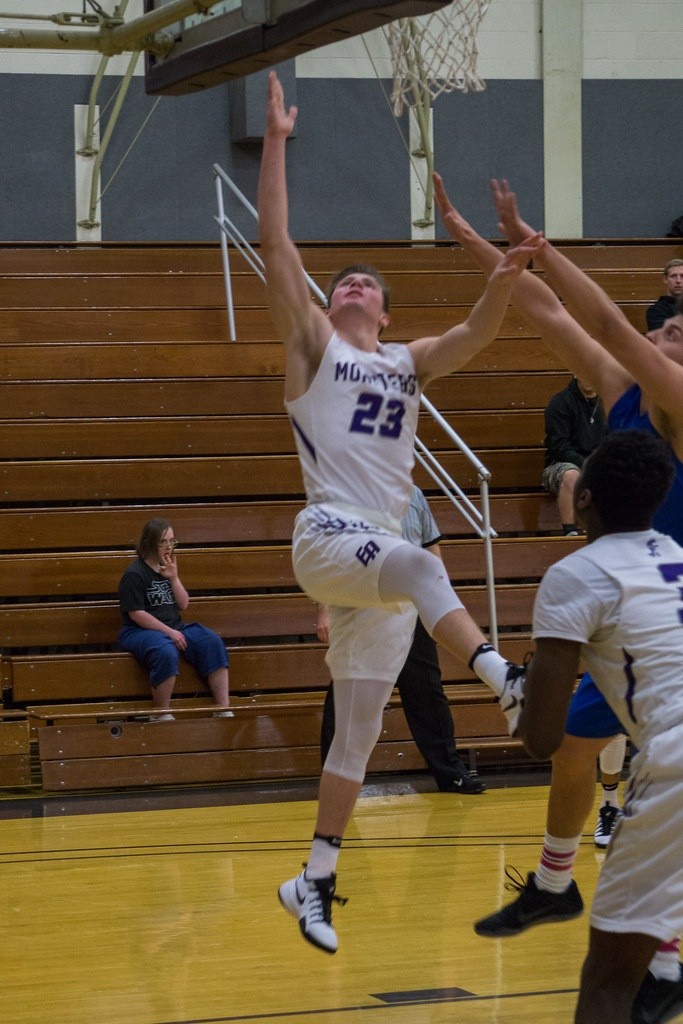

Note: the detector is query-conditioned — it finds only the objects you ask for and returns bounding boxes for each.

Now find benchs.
[0,242,683,791]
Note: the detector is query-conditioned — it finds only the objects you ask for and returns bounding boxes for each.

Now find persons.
[255,71,544,956]
[317,490,488,796]
[119,518,236,722]
[431,172,682,1024]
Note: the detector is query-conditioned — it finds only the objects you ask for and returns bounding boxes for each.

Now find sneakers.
[475,863,584,936]
[440,767,484,793]
[279,861,348,955]
[495,651,538,737]
[593,801,624,847]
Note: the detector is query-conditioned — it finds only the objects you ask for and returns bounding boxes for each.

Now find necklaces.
[589,394,601,424]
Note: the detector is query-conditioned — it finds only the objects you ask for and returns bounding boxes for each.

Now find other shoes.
[149,713,175,722]
[213,711,236,718]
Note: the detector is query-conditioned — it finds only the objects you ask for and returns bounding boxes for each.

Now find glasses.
[159,538,179,548]
